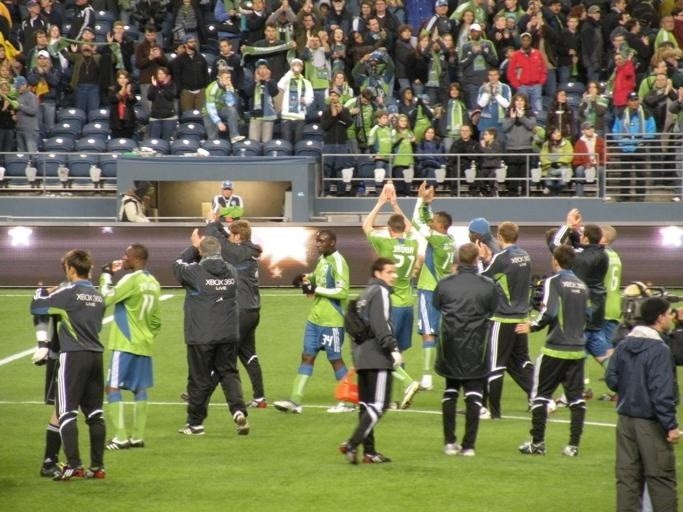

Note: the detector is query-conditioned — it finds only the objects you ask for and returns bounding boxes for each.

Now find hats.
[627,92,641,101]
[468,23,482,33]
[222,180,233,191]
[329,88,342,97]
[37,50,51,58]
[468,217,491,236]
[588,5,602,15]
[435,0,450,8]
[362,89,376,100]
[26,1,39,9]
[255,59,270,68]
[520,32,533,39]
[13,75,28,87]
[581,121,595,130]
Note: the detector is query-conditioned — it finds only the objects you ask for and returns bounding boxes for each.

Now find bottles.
[101,259,123,274]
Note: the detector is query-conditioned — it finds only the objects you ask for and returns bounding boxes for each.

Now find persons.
[1,0,683,203]
[271,178,683,465]
[210,181,244,222]
[119,180,156,222]
[604,280,683,509]
[29,213,263,484]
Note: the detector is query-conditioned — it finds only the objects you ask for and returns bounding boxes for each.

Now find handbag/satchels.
[344,299,373,346]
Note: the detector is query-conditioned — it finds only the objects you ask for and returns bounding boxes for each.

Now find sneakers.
[362,453,394,464]
[325,402,358,414]
[54,466,85,481]
[84,467,107,480]
[233,412,249,435]
[480,407,491,420]
[104,438,129,451]
[339,441,359,465]
[518,442,546,456]
[523,387,594,413]
[40,464,62,476]
[458,447,476,457]
[129,437,145,448]
[246,397,267,408]
[598,394,618,402]
[561,446,580,458]
[401,381,422,410]
[273,400,303,415]
[444,441,460,455]
[177,425,205,436]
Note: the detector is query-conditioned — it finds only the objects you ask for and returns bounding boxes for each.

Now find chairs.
[0,1,611,196]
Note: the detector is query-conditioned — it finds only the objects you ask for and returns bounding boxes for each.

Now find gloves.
[389,345,404,370]
[301,283,316,295]
[291,274,306,287]
